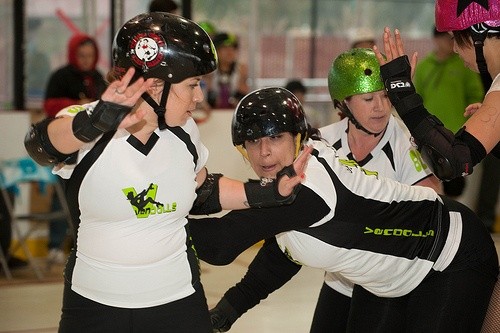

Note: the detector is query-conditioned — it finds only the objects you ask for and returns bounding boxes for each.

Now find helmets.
[111,10,218,84]
[230,86,309,148]
[327,47,389,109]
[212,32,239,48]
[434,0,500,41]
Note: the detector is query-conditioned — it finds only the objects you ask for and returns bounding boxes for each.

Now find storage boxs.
[10,228,69,262]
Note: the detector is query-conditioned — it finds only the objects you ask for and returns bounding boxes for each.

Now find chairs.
[0,158,75,282]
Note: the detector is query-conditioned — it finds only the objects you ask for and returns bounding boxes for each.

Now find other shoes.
[0,254,28,271]
[47,248,65,264]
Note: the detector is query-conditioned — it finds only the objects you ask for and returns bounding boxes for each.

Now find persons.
[189,87,500,333]
[310,1,499,333]
[24,12,312,333]
[44,20,307,264]
[0,184,30,270]
